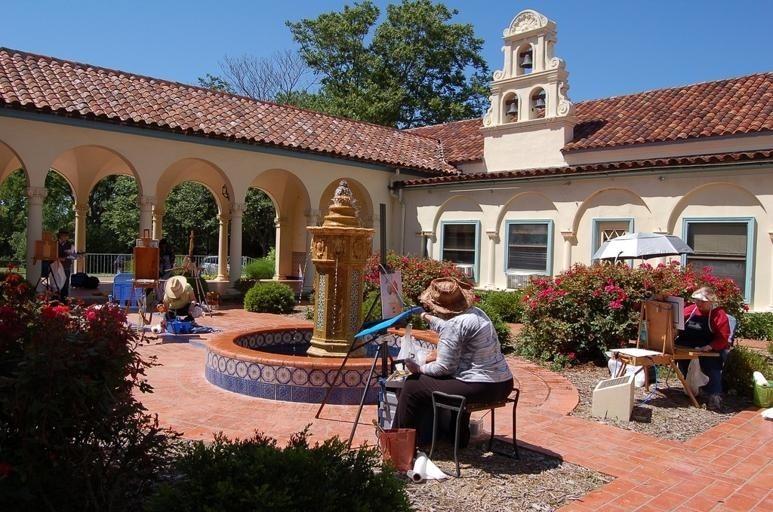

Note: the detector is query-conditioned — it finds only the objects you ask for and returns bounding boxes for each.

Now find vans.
[113,255,171,277]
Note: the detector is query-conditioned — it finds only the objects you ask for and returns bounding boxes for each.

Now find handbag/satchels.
[71,272,87,288]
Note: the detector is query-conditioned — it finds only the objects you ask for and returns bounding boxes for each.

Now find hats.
[58,228,70,235]
[417,277,473,316]
[164,275,187,298]
[691,287,714,301]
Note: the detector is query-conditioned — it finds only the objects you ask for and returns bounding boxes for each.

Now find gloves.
[409,304,427,320]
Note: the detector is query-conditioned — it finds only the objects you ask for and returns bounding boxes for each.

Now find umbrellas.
[591,231,696,289]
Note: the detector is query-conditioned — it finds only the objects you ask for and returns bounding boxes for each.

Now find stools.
[430,386,520,476]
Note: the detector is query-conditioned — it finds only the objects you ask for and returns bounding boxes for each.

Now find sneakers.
[708,395,721,412]
[454,428,471,449]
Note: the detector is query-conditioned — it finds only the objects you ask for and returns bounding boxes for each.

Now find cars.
[199,256,246,273]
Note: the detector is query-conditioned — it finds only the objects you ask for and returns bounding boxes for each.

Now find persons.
[55,228,75,304]
[159,239,175,278]
[391,277,513,448]
[677,286,731,406]
[163,276,198,321]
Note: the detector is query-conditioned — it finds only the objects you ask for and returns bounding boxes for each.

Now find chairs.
[665,313,736,399]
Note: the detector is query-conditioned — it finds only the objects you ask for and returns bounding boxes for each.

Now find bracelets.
[420,311,428,320]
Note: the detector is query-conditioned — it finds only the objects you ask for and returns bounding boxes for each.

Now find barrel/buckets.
[753,380,773,408]
[377,428,416,475]
